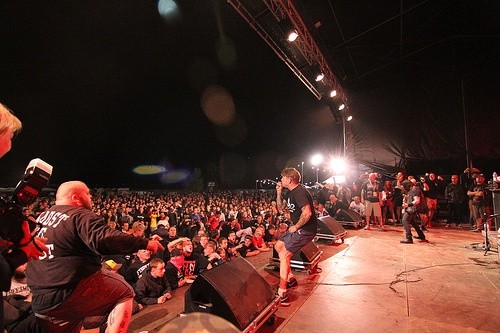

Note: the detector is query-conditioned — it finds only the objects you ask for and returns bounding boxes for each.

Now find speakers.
[338,209,364,222]
[317,215,346,235]
[273,240,321,262]
[185,255,281,333]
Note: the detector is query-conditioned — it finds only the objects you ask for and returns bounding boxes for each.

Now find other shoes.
[413,236,425,239]
[377,225,382,231]
[275,291,290,306]
[400,239,413,243]
[274,277,298,289]
[364,225,370,230]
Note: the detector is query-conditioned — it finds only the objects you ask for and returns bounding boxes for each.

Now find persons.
[0,102,500,333]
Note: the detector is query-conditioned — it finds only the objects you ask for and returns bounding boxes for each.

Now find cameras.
[0,158,54,243]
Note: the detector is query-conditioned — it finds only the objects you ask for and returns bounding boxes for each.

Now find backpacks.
[416,187,426,210]
